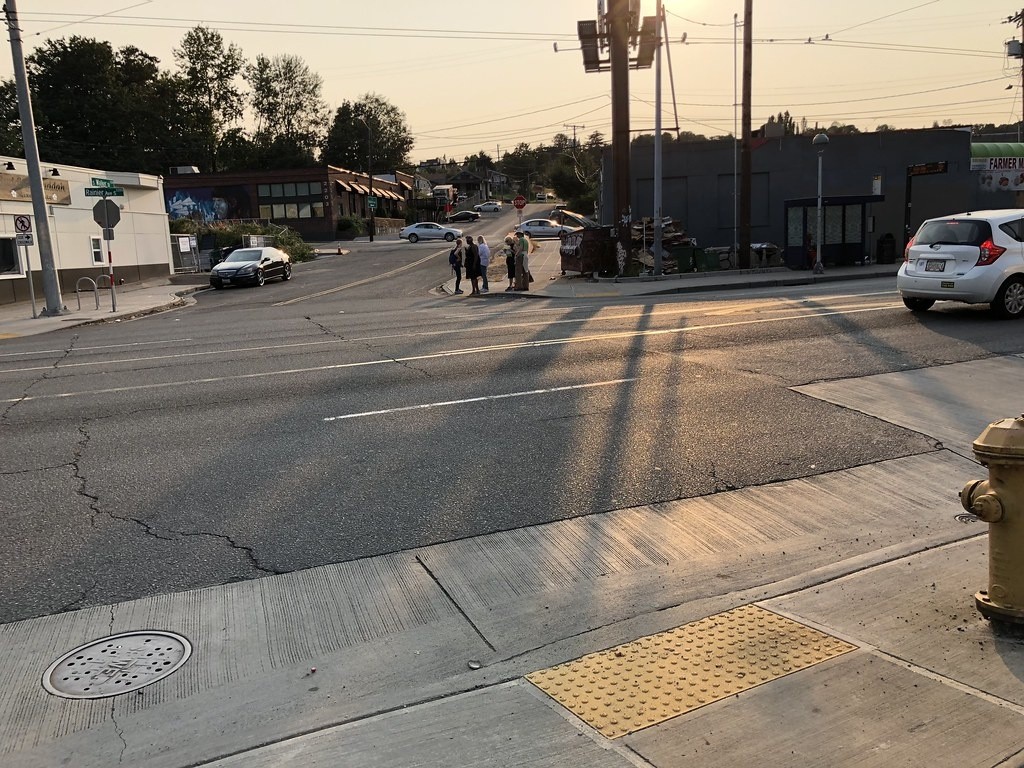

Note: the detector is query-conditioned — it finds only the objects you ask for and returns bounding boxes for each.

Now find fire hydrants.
[958,413,1024,629]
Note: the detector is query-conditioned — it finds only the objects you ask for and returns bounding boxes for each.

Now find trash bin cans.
[876,232,896,264]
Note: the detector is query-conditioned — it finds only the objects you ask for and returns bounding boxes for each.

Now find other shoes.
[455,289,463,294]
[514,287,528,291]
[505,287,512,291]
[469,291,480,295]
[480,288,489,292]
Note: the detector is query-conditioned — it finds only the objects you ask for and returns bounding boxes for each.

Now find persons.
[452,239,464,294]
[503,232,534,292]
[465,236,481,295]
[478,235,490,292]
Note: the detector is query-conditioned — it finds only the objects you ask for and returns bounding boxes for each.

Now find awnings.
[400,181,412,190]
[367,187,382,197]
[384,190,397,200]
[392,192,404,201]
[348,181,364,194]
[336,180,351,191]
[358,184,373,195]
[376,188,390,199]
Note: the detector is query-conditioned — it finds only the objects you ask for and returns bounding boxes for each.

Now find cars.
[512,218,574,239]
[209,246,292,291]
[398,221,463,243]
[473,201,502,212]
[445,210,481,224]
[895,207,1024,320]
[536,192,547,203]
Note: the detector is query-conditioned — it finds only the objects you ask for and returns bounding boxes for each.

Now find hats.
[514,230,523,235]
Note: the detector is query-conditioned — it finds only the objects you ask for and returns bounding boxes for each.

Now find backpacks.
[449,247,457,263]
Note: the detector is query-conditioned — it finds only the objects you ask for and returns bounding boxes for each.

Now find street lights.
[354,116,373,242]
[812,131,829,275]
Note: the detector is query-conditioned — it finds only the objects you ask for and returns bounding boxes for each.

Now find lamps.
[49,168,60,176]
[4,162,16,170]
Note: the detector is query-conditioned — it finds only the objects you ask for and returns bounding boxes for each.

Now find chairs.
[933,229,958,243]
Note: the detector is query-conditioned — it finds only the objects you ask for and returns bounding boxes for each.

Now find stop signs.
[513,195,527,209]
